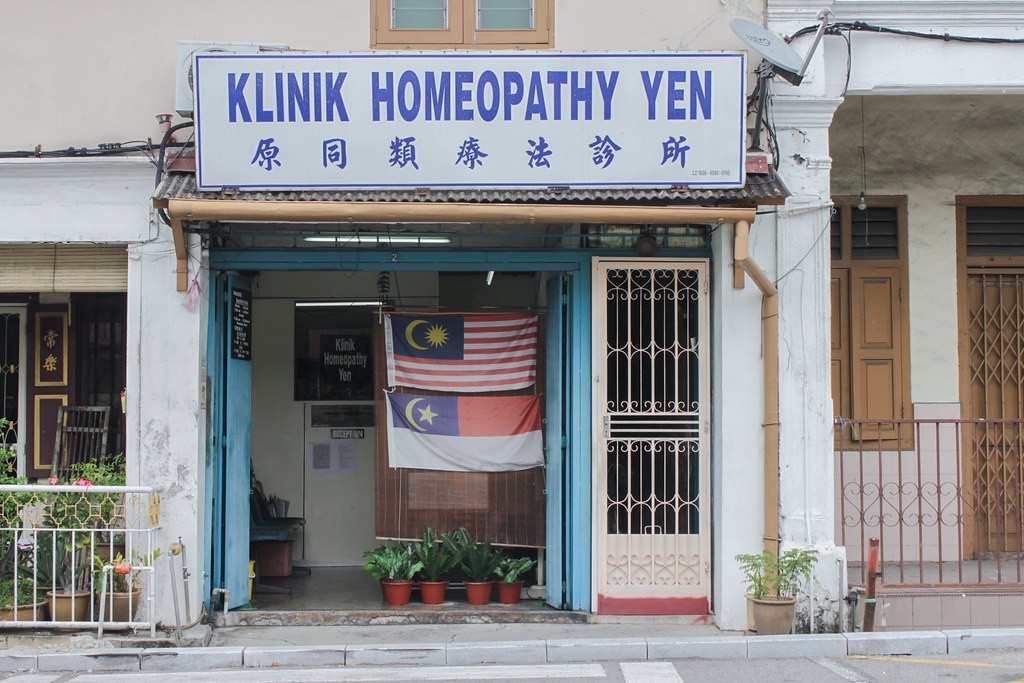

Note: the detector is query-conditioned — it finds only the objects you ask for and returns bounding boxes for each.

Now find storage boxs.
[251,540,295,578]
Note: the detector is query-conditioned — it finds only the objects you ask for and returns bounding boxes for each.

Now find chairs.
[222,445,311,595]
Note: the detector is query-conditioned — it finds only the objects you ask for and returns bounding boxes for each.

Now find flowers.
[41,451,129,589]
[88,545,163,594]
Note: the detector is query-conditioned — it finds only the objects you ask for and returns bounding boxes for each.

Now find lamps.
[858,191,867,211]
[302,230,452,244]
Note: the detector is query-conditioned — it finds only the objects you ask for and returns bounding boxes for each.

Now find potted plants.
[441,527,509,605]
[88,532,125,561]
[392,527,478,603]
[0,578,49,631]
[493,556,538,603]
[364,539,426,604]
[737,548,820,635]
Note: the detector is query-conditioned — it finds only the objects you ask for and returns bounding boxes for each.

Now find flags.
[382,389,545,472]
[385,313,539,392]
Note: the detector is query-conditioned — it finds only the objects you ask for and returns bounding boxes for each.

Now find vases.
[96,589,141,632]
[48,589,90,635]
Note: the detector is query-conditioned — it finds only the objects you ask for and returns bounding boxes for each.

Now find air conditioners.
[175,41,289,121]
